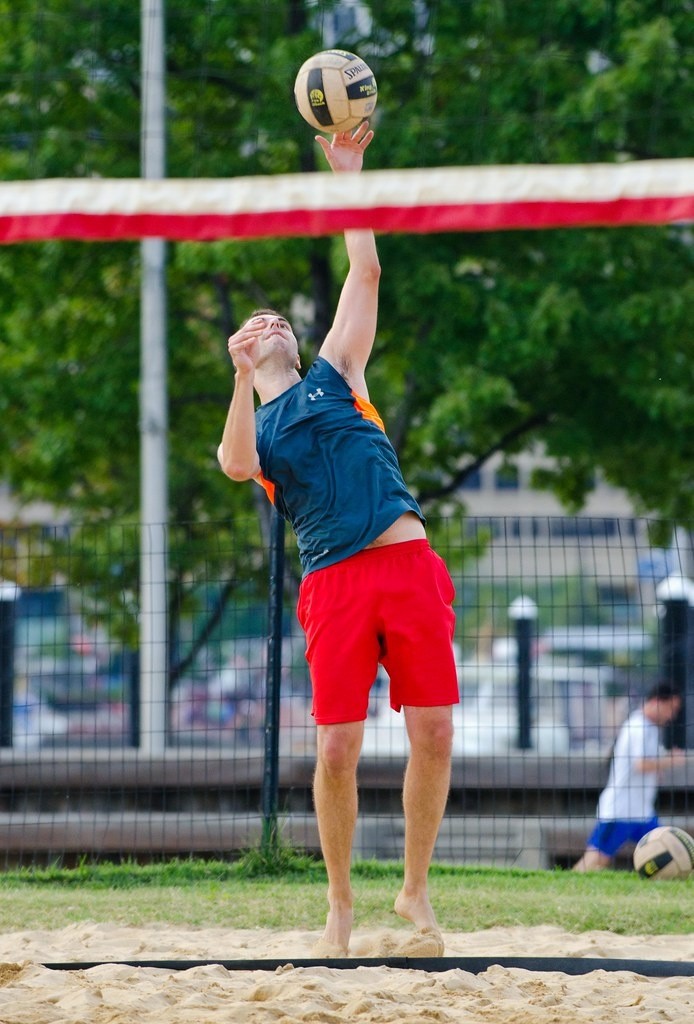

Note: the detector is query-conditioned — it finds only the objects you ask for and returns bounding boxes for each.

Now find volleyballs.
[293,50,377,132]
[633,825,693,880]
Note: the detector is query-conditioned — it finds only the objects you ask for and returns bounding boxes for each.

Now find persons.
[565,680,685,877]
[215,119,458,965]
